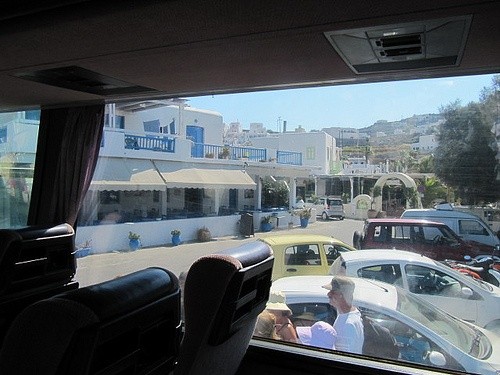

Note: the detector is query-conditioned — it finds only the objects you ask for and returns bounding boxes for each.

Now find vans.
[400,208,500,251]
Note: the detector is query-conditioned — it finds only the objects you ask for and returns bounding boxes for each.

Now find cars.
[355,215,480,262]
[269,273,500,375]
[312,197,345,220]
[254,232,359,281]
[331,247,500,337]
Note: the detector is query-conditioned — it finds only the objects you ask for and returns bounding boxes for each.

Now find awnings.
[87,157,257,191]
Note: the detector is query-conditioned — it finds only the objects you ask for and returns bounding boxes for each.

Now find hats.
[295,321,337,350]
[321,277,356,295]
[266,291,293,318]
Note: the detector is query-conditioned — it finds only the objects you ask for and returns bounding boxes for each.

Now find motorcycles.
[448,246,500,289]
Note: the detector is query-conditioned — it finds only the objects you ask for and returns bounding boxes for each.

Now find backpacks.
[361,313,400,362]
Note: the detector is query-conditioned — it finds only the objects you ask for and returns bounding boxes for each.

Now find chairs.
[0,225,274,375]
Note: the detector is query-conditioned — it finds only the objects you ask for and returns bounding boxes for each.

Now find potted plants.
[127,231,140,251]
[262,215,273,232]
[171,230,180,246]
[75,240,93,258]
[299,207,311,228]
[221,148,229,159]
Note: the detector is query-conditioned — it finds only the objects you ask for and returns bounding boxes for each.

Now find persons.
[252,276,364,354]
[296,196,304,203]
